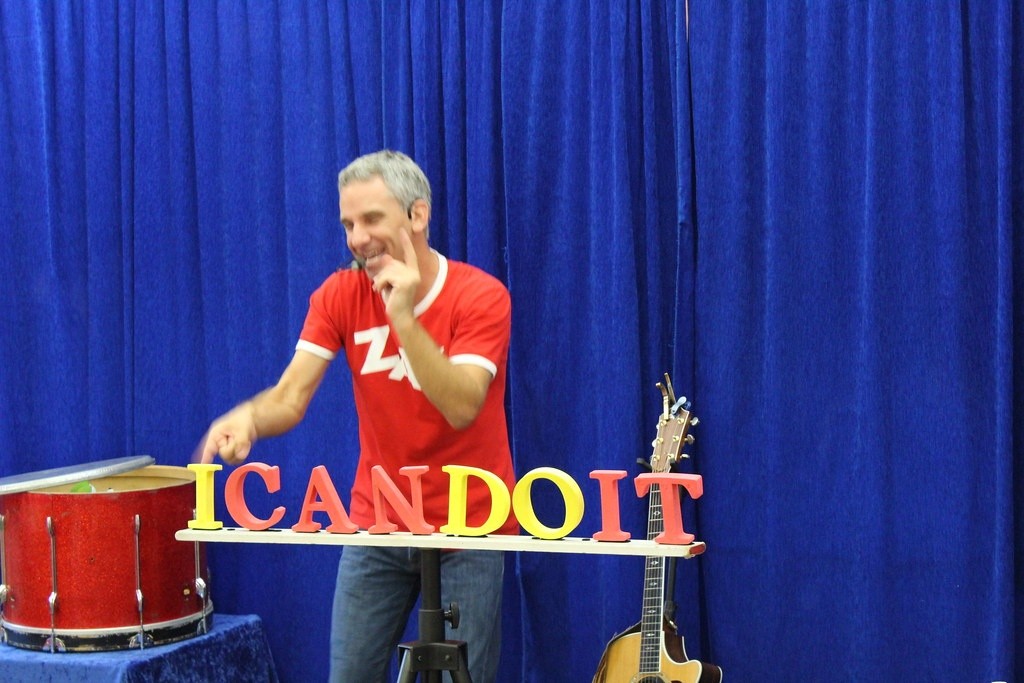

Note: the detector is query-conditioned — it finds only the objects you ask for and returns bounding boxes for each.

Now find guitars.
[592,371,722,683]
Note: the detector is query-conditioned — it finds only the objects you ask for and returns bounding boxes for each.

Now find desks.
[0,612,279,683]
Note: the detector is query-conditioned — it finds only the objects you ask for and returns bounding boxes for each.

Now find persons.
[190,151,517,683]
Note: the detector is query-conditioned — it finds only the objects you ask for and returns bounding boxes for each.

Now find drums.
[0,455,214,652]
